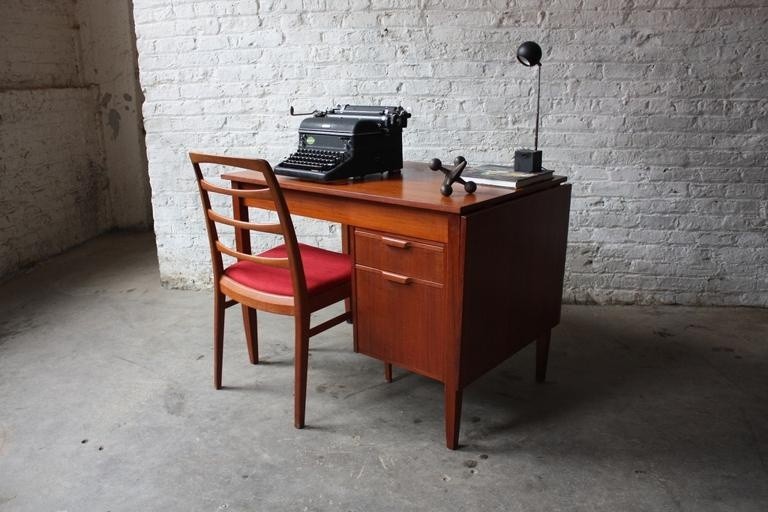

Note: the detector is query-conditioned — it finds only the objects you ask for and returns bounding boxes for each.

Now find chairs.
[189,152,391,429]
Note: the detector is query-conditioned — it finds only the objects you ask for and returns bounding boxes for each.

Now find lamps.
[514,41,542,172]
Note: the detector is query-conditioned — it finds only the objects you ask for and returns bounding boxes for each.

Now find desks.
[221,162,572,450]
